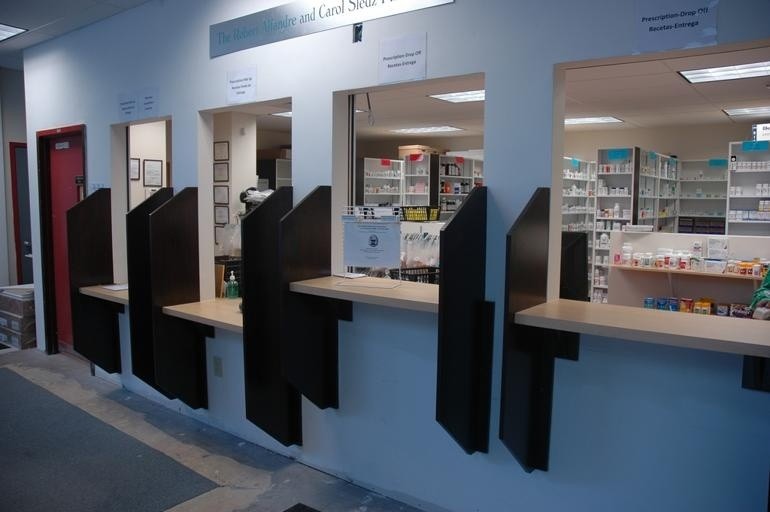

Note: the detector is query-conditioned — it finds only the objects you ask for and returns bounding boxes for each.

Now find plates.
[257,157,291,197]
[358,155,487,222]
[559,136,770,319]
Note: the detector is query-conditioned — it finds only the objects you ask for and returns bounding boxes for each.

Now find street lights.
[223,224,241,250]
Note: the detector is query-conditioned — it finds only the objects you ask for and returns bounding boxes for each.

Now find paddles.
[1,367,222,511]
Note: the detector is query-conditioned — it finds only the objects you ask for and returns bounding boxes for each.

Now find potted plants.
[213,141,230,244]
[143,159,163,188]
[129,157,141,183]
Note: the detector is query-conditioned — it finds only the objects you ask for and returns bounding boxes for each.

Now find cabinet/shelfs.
[562,160,770,318]
[440,161,469,211]
